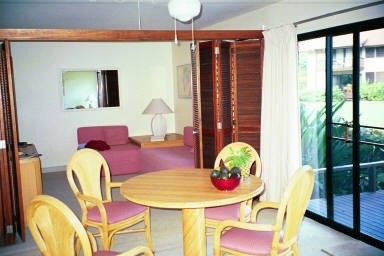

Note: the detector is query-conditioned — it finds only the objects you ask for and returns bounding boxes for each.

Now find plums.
[210,166,241,179]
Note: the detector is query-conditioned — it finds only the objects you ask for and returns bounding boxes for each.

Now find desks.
[120,168,265,256]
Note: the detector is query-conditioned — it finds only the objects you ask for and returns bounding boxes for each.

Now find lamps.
[168,0,201,51]
[142,99,174,137]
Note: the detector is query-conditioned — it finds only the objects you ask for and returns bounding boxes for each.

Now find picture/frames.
[176,64,192,97]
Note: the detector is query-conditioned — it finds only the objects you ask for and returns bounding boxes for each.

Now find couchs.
[78,126,140,176]
[141,127,195,173]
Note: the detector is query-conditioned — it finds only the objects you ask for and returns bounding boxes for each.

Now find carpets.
[321,240,384,256]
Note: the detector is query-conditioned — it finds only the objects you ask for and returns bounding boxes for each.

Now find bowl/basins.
[210,175,241,191]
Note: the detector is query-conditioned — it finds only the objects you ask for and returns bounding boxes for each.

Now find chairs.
[25,195,154,256]
[66,148,155,253]
[205,142,261,237]
[214,165,315,256]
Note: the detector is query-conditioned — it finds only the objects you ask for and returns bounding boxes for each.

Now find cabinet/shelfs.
[19,144,43,228]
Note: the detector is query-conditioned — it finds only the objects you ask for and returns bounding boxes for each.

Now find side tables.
[128,133,184,148]
